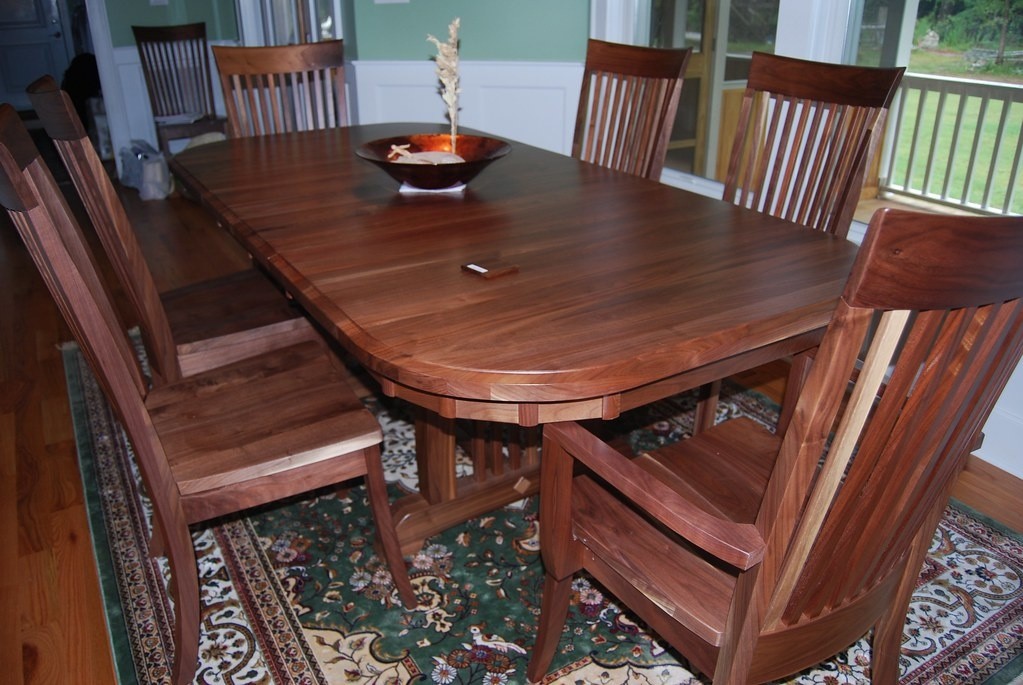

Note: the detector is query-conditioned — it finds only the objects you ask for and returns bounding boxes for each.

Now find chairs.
[1,20,1023,684]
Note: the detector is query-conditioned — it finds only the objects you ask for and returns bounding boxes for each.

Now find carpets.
[59,323,1023,685]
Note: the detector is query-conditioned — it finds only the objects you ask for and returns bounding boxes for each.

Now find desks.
[166,122,863,684]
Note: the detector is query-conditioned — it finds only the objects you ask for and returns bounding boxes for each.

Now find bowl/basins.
[354,132,512,191]
